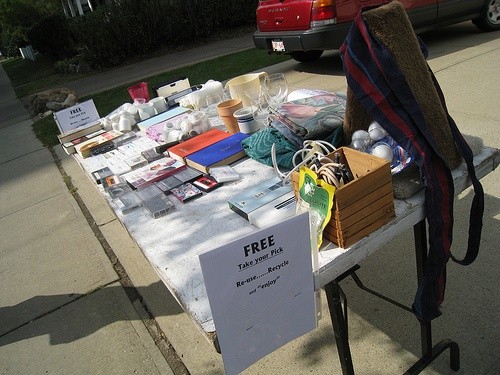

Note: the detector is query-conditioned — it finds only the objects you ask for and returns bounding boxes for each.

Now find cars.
[252,0,500,64]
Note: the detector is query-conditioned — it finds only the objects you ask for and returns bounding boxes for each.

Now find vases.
[216,98,243,134]
[232,106,259,134]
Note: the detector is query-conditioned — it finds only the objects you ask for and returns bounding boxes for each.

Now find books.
[186,131,252,177]
[167,127,232,165]
[57,121,125,159]
[136,107,193,133]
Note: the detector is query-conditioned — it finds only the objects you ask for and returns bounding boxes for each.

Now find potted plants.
[11,25,36,63]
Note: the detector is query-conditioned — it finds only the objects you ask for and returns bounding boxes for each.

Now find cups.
[217,99,242,133]
[233,106,261,134]
[103,96,167,132]
[128,82,150,105]
[264,72,289,107]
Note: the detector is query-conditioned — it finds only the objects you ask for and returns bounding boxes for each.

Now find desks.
[247,146,500,375]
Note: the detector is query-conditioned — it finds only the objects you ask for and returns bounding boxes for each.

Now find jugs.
[224,70,271,108]
